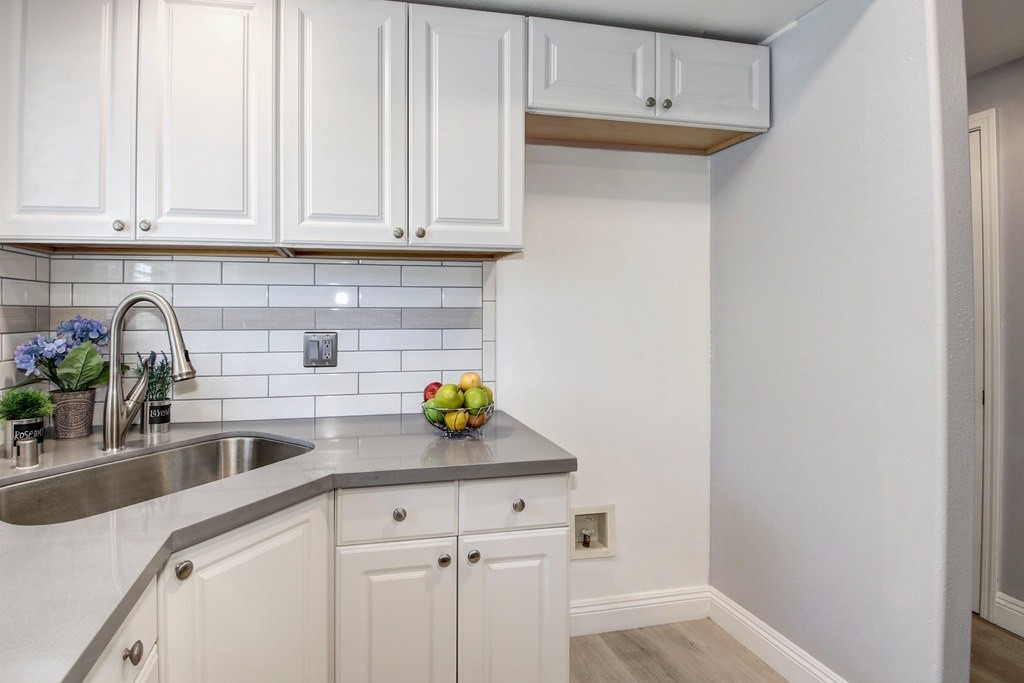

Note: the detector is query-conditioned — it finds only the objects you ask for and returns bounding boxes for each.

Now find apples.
[423,373,493,427]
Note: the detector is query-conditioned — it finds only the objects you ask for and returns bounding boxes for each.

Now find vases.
[46,388,94,440]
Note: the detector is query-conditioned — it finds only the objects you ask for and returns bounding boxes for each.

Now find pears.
[444,409,469,431]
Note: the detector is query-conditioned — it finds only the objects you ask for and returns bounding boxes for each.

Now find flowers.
[15,313,129,389]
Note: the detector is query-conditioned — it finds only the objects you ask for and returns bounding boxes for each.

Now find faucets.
[104,290,194,451]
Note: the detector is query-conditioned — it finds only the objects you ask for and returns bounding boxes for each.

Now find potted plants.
[131,350,173,436]
[0,388,58,458]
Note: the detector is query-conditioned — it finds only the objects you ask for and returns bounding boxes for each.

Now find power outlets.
[303,331,337,367]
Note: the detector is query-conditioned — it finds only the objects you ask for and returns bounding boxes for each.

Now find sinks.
[0,430,316,526]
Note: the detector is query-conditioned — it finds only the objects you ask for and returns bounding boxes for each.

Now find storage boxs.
[3,3,290,256]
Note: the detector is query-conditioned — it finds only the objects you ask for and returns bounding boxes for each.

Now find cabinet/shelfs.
[158,491,332,680]
[335,470,573,680]
[77,571,159,683]
[525,16,775,157]
[281,2,524,260]
